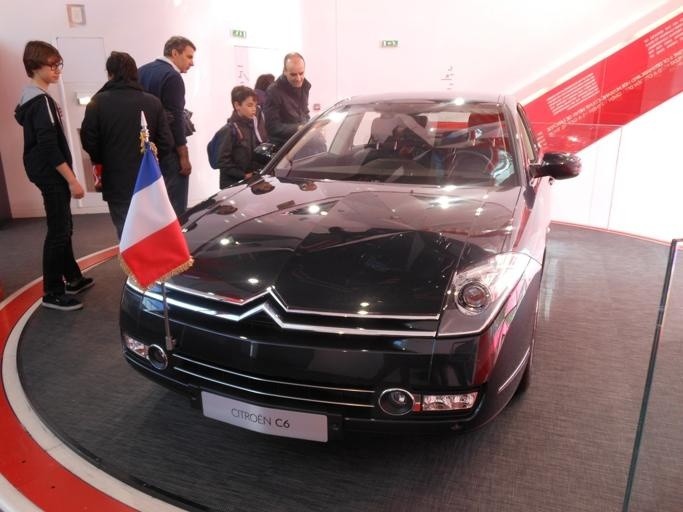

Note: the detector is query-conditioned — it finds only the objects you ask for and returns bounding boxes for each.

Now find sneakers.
[41,277,95,311]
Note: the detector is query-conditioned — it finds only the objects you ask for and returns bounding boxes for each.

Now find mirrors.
[54,31,127,211]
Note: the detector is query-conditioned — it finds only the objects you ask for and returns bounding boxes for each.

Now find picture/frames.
[66,4,86,26]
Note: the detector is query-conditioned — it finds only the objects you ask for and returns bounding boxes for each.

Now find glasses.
[42,61,64,71]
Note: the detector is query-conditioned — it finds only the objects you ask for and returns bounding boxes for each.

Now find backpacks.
[206,122,244,169]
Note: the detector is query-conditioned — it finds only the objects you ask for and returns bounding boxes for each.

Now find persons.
[79,51,175,240]
[14,41,94,311]
[250,72,275,141]
[216,86,269,190]
[138,36,196,221]
[263,52,310,161]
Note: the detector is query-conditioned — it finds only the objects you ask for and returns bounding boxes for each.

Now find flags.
[114,145,193,290]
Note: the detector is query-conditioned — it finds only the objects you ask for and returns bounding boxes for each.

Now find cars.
[120,94,582,443]
